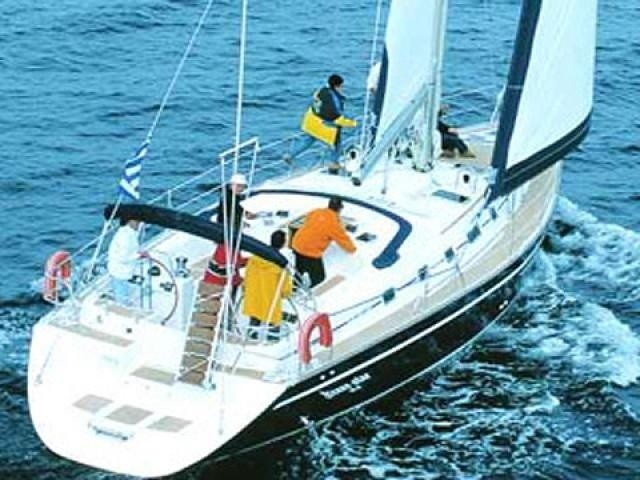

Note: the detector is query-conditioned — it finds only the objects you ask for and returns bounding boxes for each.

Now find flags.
[116,140,150,200]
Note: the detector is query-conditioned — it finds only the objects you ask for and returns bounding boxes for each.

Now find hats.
[328,197,344,208]
[229,173,247,185]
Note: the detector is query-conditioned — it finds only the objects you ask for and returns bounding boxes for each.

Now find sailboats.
[28,0,597,477]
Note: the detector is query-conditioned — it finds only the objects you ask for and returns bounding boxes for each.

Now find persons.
[107,216,148,306]
[218,174,258,233]
[242,229,292,342]
[301,74,363,176]
[204,233,249,330]
[438,104,476,158]
[288,198,356,295]
